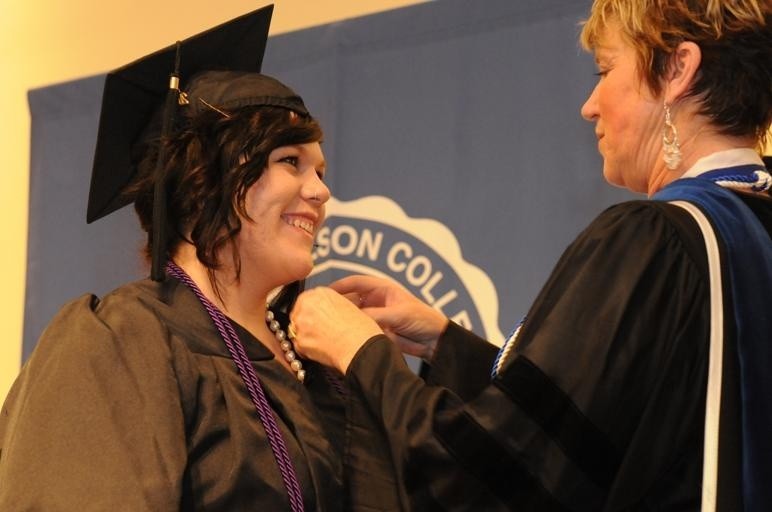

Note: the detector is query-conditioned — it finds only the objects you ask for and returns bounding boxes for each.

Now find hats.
[86,4,310,225]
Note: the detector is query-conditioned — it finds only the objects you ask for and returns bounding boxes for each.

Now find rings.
[359,294,363,306]
[285,323,297,338]
[285,0,772,511]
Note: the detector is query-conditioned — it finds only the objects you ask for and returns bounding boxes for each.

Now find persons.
[0,5,398,510]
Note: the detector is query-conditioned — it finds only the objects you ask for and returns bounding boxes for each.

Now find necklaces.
[266,309,309,384]
